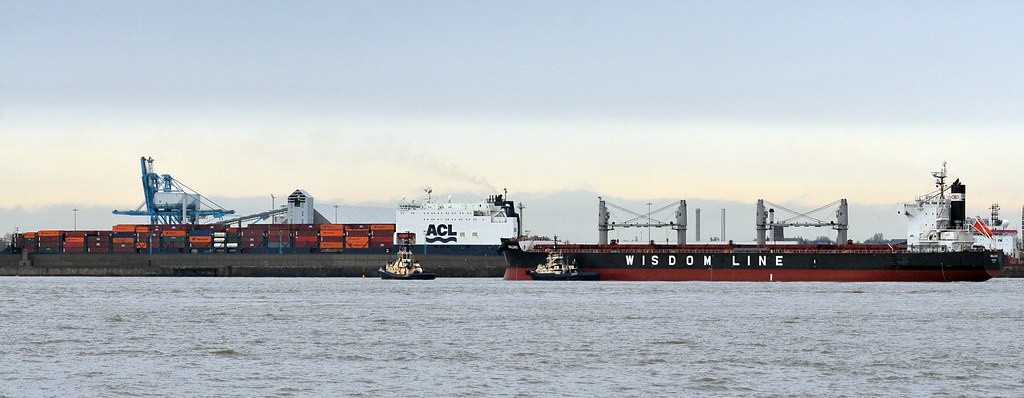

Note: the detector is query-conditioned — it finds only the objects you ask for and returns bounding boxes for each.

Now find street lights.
[73,208,78,229]
[271,194,275,225]
[334,205,339,222]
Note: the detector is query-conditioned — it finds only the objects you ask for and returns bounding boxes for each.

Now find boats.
[529,235,600,279]
[377,244,436,279]
[504,164,1001,282]
